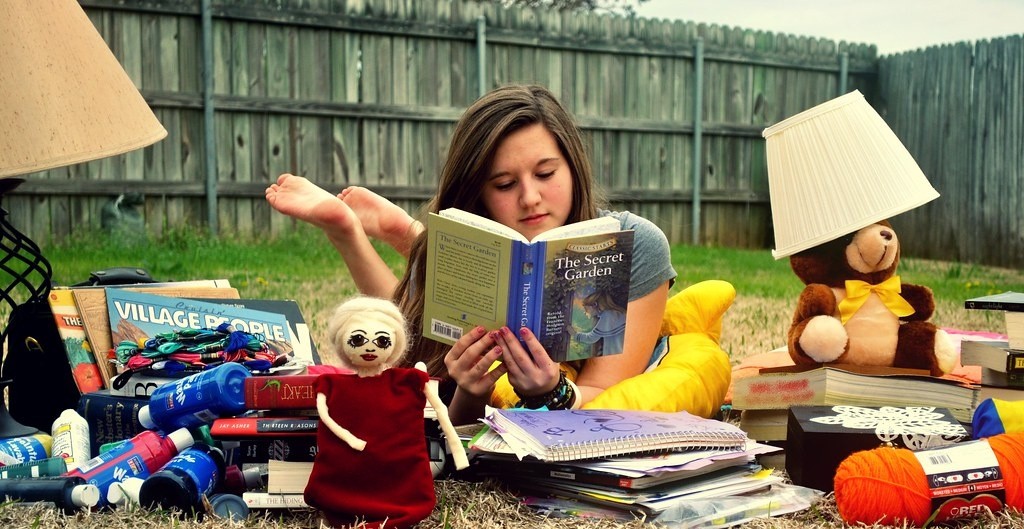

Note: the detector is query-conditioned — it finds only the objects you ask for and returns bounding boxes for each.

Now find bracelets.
[514,372,582,412]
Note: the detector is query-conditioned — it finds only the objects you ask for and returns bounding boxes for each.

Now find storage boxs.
[786,404,969,495]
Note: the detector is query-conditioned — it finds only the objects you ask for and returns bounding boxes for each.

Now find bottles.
[0,409,261,521]
[138,362,252,431]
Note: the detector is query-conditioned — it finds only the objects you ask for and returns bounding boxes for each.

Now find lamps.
[1,0,168,440]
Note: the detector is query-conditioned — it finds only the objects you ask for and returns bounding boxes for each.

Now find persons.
[266,86,676,425]
[302,296,468,529]
[566,292,627,355]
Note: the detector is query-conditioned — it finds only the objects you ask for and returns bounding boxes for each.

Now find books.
[465,402,780,519]
[423,208,634,362]
[738,291,1024,471]
[210,372,442,508]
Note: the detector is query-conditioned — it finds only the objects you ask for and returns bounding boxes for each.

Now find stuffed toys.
[787,219,959,378]
